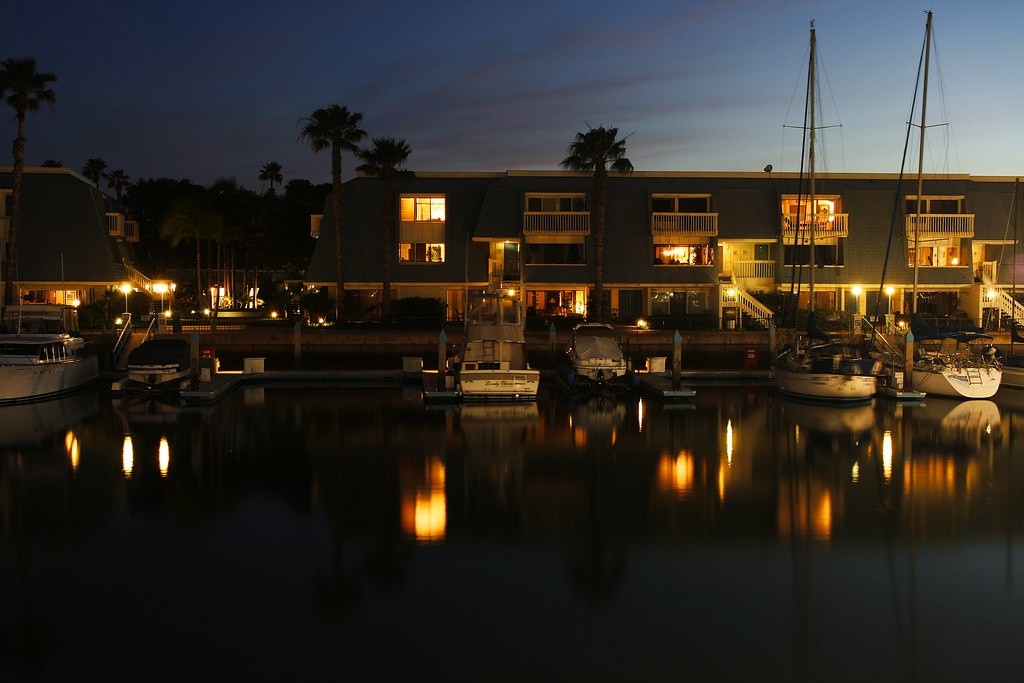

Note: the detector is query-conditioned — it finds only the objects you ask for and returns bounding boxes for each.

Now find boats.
[462,398,539,473]
[1,291,100,408]
[567,321,627,384]
[0,381,104,452]
[126,338,195,390]
[459,293,540,401]
[127,376,192,413]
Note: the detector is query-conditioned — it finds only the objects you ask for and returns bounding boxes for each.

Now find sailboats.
[885,8,1002,400]
[773,394,1015,683]
[770,19,881,405]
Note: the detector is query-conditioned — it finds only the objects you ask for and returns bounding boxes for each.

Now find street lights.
[122,285,130,313]
[157,285,166,312]
